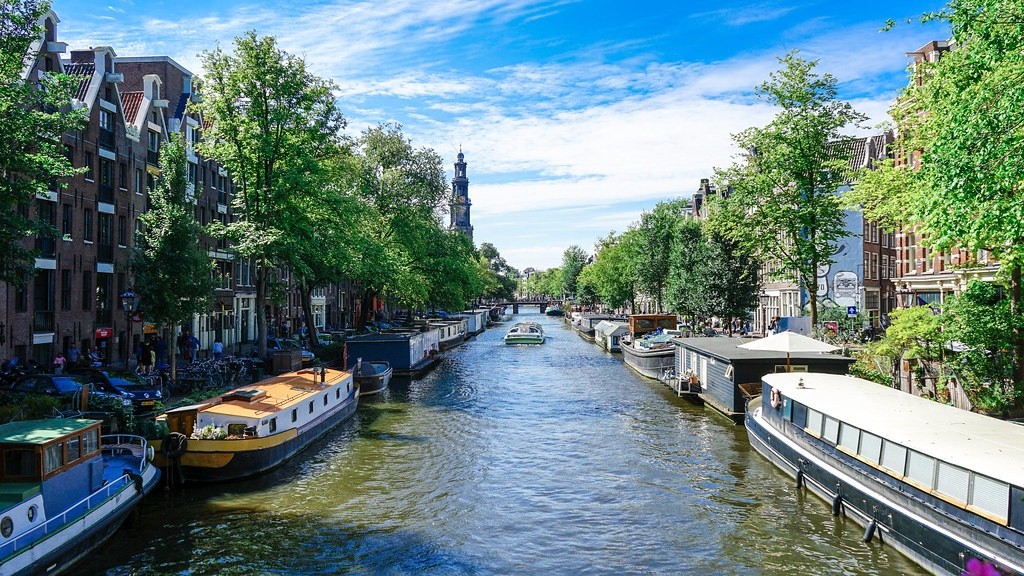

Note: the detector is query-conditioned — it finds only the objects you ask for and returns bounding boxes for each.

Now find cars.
[0,373,133,420]
[64,367,162,414]
[316,333,334,346]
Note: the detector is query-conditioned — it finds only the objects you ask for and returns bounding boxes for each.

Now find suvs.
[250,336,316,366]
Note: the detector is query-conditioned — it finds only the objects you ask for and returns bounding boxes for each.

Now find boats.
[545,305,564,316]
[0,417,164,576]
[563,308,630,353]
[344,356,393,398]
[325,305,506,378]
[619,311,680,379]
[661,329,858,425]
[503,321,546,345]
[132,365,361,487]
[738,371,1024,576]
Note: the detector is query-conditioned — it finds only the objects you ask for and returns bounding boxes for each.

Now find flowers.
[190,417,228,441]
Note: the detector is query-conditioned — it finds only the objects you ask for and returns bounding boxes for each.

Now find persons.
[769,316,775,331]
[732,318,750,334]
[53,354,67,372]
[176,330,201,364]
[85,342,104,367]
[135,336,167,373]
[67,342,80,370]
[281,321,289,338]
[212,339,223,360]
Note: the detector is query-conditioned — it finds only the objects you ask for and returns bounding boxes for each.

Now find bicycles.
[138,354,248,403]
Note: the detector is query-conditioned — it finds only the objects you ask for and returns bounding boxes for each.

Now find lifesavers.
[160,432,188,461]
[769,388,781,409]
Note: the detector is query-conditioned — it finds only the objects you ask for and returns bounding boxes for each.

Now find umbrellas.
[735,329,841,372]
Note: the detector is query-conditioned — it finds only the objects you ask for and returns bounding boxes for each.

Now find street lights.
[121,289,137,371]
